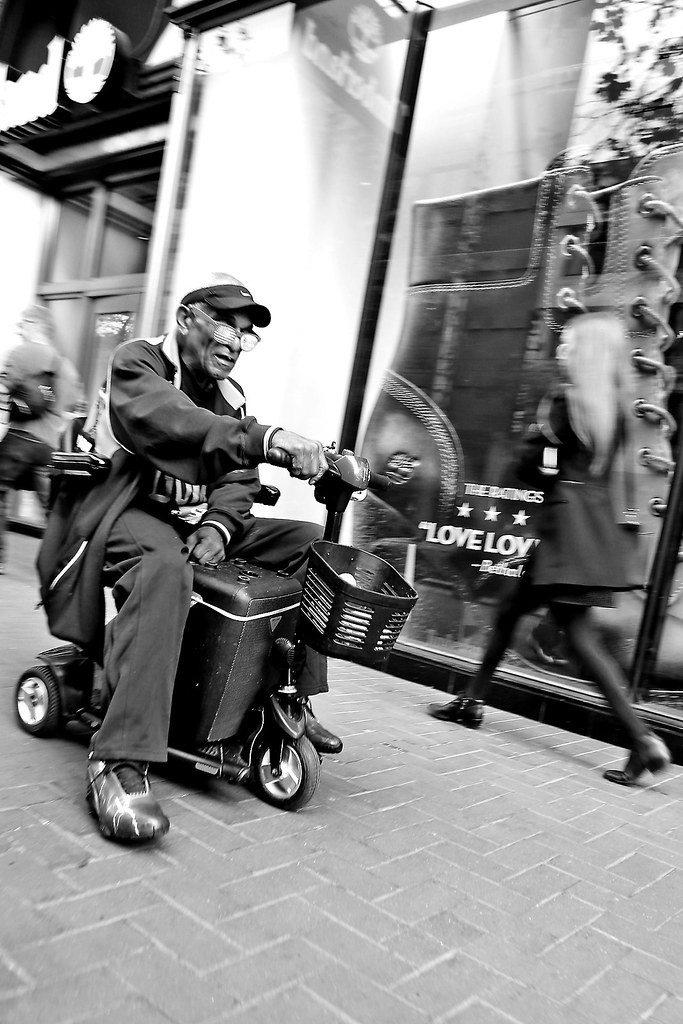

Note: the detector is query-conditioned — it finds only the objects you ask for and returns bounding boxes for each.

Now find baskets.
[295,540,419,664]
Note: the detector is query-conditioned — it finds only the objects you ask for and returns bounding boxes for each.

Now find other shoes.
[86,752,170,844]
[525,631,569,665]
[297,696,342,753]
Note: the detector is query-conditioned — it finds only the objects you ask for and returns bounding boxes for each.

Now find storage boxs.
[297,538,419,667]
[173,555,302,741]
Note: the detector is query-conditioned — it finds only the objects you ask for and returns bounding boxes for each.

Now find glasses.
[188,304,261,352]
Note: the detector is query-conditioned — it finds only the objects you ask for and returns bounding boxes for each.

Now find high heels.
[605,732,671,785]
[427,690,485,728]
[350,145,683,690]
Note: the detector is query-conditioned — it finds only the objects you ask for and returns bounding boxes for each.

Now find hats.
[178,272,271,328]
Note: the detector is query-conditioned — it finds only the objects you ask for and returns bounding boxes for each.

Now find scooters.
[15,441,421,813]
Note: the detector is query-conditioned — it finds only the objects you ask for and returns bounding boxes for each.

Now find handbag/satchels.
[10,368,55,423]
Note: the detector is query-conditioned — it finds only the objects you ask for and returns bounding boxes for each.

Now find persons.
[0,304,84,578]
[32,273,333,845]
[426,309,672,787]
